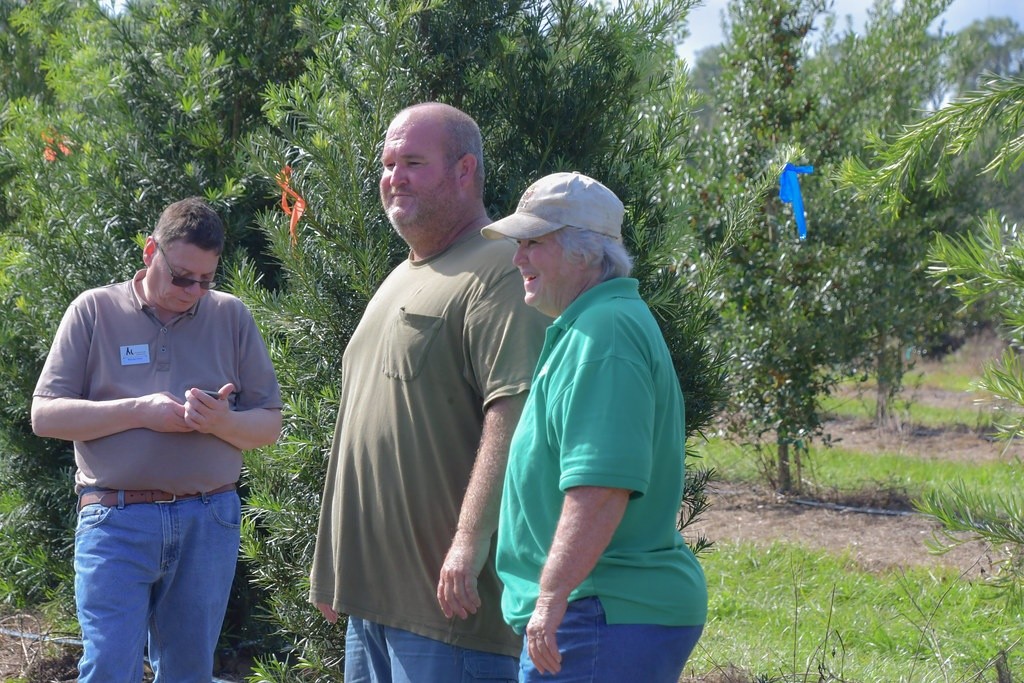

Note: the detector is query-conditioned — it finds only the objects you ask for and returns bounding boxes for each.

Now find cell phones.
[202,389,220,400]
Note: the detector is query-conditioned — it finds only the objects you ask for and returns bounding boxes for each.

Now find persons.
[31,199,284,683]
[309,102,555,683]
[481,171,708,683]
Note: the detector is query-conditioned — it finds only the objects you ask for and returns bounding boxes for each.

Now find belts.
[80,483,236,510]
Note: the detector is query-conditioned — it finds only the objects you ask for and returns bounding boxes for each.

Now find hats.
[481,171,625,240]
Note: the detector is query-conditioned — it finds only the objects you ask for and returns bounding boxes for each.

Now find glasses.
[152,236,225,290]
